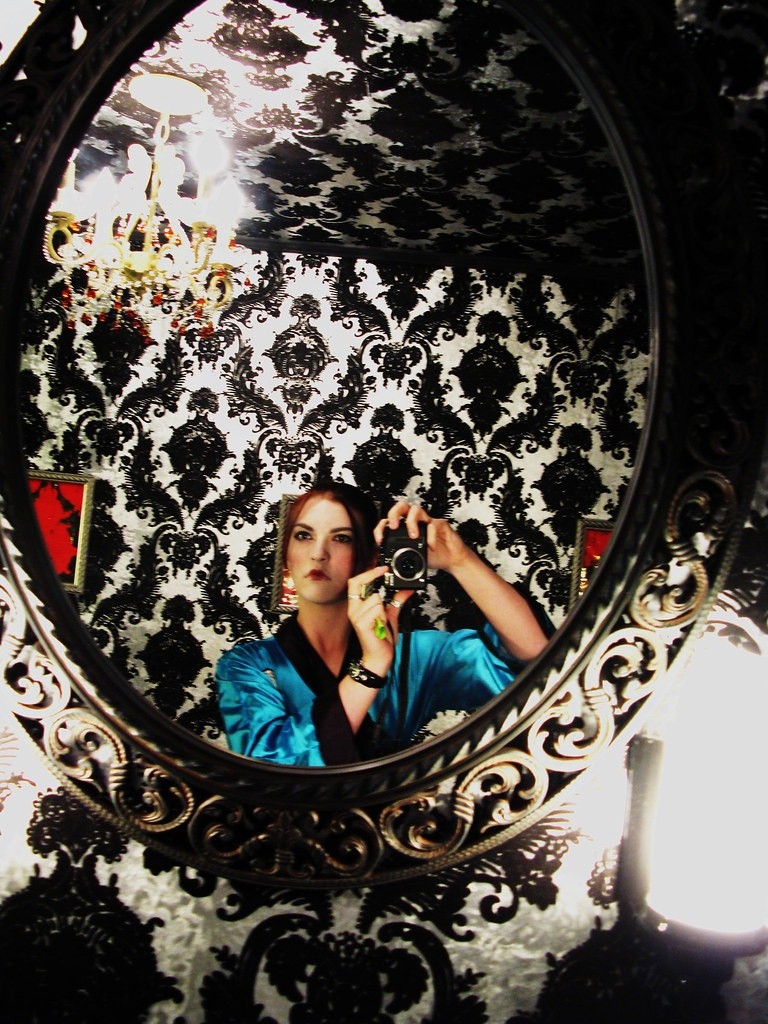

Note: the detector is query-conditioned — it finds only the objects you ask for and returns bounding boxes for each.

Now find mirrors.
[0,0,768,887]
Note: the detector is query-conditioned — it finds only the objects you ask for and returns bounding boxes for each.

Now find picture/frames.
[569,518,616,616]
[23,470,95,592]
[270,494,382,615]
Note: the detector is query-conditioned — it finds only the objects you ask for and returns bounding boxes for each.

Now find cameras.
[380,518,429,591]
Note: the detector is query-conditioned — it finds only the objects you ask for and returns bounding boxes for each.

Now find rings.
[347,595,361,600]
[388,600,403,609]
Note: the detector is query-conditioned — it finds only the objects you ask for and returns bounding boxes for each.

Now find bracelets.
[344,664,389,690]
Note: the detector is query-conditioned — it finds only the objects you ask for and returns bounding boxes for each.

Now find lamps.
[42,74,255,340]
[532,611,768,1024]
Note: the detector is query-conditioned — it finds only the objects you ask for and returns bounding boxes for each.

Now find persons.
[213,482,551,770]
